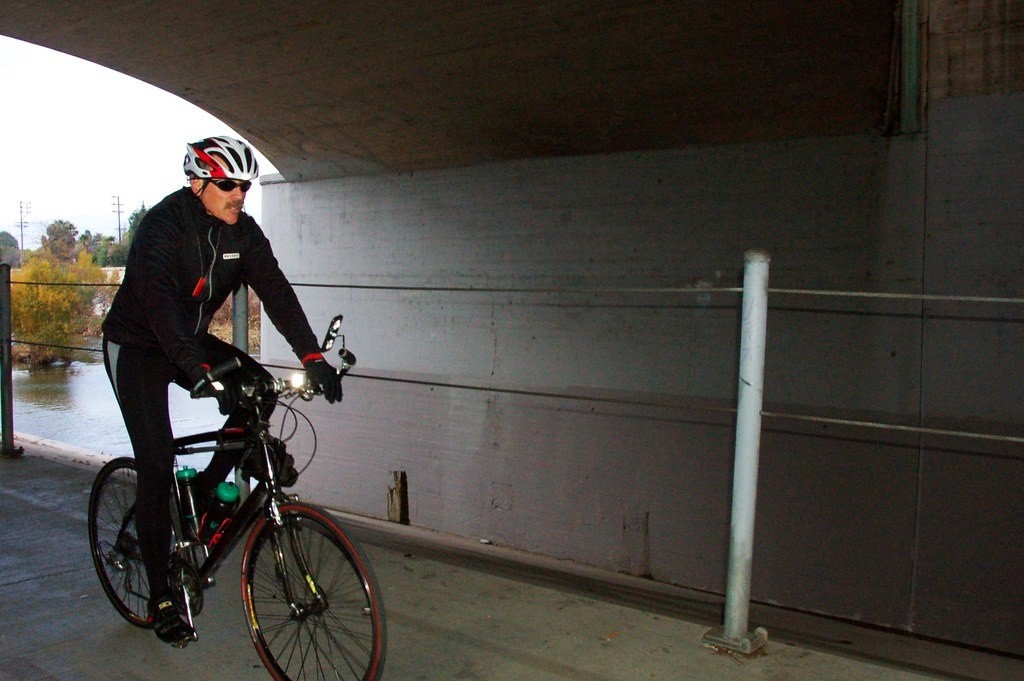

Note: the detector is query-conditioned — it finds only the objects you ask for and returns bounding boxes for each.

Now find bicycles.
[86,312,393,681]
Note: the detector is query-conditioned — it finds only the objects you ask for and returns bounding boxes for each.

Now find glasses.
[197,177,252,194]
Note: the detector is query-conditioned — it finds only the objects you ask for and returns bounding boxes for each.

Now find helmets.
[183,135,259,181]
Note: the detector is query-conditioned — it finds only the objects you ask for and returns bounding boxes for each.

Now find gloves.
[305,360,343,405]
[205,379,251,416]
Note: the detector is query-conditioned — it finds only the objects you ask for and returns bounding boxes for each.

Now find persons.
[101,137,344,649]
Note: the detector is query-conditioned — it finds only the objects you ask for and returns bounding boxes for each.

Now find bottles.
[198,481,240,546]
[175,465,204,542]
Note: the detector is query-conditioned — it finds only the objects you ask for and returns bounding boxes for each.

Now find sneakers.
[148,594,194,643]
[193,473,218,512]
